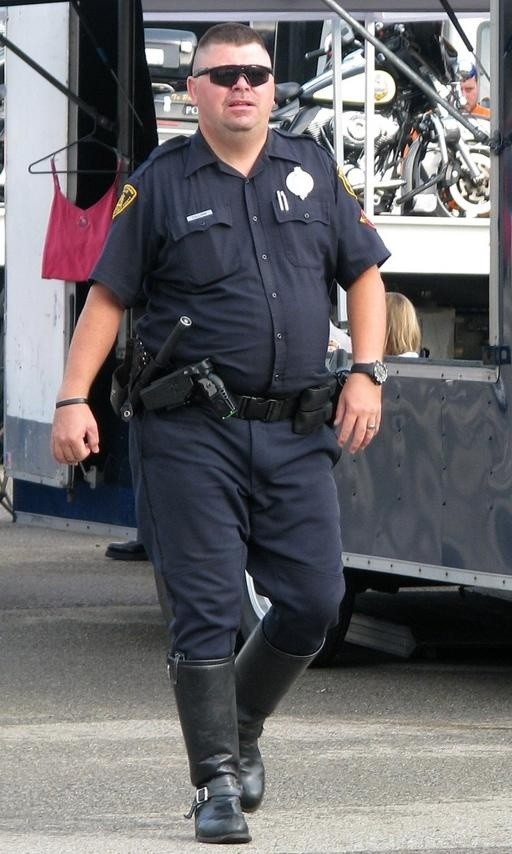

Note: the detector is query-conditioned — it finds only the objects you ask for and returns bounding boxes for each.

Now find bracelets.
[53,396,90,409]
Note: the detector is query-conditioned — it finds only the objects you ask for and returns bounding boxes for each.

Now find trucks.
[4,4,512,605]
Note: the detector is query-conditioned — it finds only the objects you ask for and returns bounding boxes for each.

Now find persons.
[48,21,395,843]
[402,59,491,216]
[383,291,422,357]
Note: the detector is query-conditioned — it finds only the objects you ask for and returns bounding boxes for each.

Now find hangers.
[26,108,139,171]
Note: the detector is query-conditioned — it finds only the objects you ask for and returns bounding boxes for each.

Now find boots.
[167,650,252,843]
[234,615,326,812]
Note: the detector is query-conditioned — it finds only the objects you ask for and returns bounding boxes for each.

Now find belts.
[191,390,298,423]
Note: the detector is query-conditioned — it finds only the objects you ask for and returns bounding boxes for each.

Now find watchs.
[350,359,390,385]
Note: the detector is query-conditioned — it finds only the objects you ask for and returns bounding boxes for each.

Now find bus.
[143,18,491,147]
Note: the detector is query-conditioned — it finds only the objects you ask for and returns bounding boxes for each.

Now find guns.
[141,356,238,420]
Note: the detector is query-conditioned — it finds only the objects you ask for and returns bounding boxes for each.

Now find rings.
[368,423,376,430]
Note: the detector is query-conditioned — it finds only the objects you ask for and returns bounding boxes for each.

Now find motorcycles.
[268,21,490,219]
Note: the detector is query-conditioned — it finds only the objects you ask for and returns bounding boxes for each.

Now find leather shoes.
[106,540,148,560]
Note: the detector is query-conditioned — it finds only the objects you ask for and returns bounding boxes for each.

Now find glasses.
[194,65,273,87]
